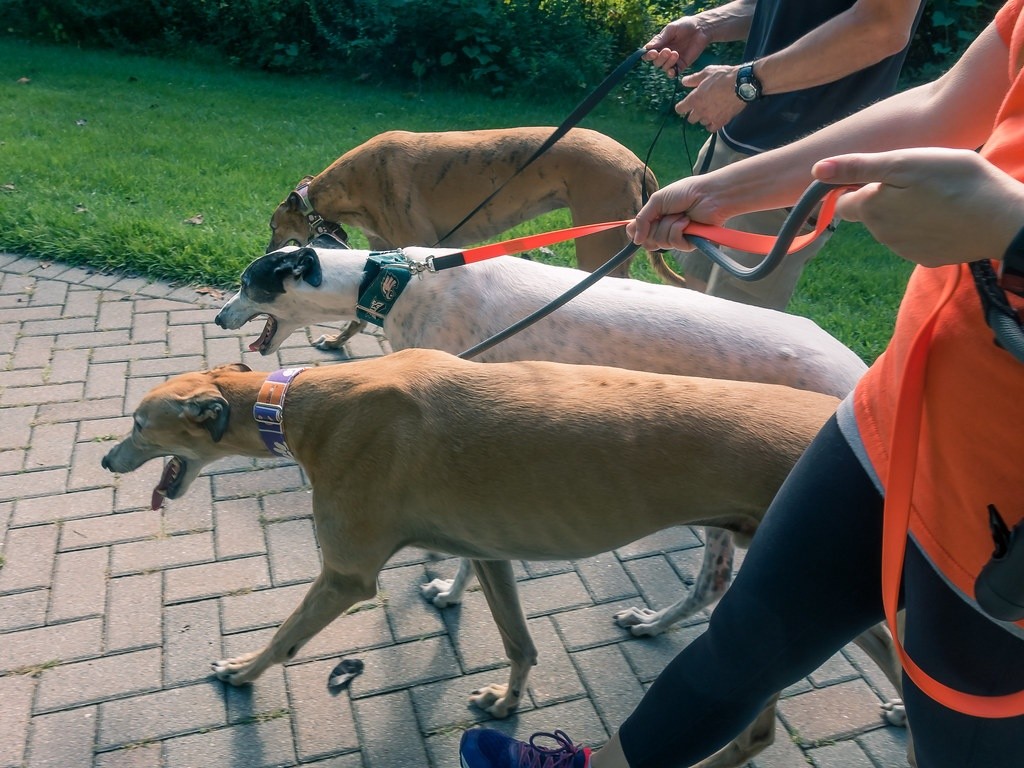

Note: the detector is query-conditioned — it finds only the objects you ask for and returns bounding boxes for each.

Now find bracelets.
[997,227,1024,299]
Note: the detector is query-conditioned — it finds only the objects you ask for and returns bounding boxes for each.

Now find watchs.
[734,60,762,104]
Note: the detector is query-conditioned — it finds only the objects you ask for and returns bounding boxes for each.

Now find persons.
[459,0,1024,768]
[642,0,927,314]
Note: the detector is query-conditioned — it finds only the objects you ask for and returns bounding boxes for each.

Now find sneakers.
[459,730,591,768]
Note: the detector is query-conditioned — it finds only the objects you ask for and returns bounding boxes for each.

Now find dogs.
[213,245,869,399]
[101,349,908,768]
[266,127,690,287]
